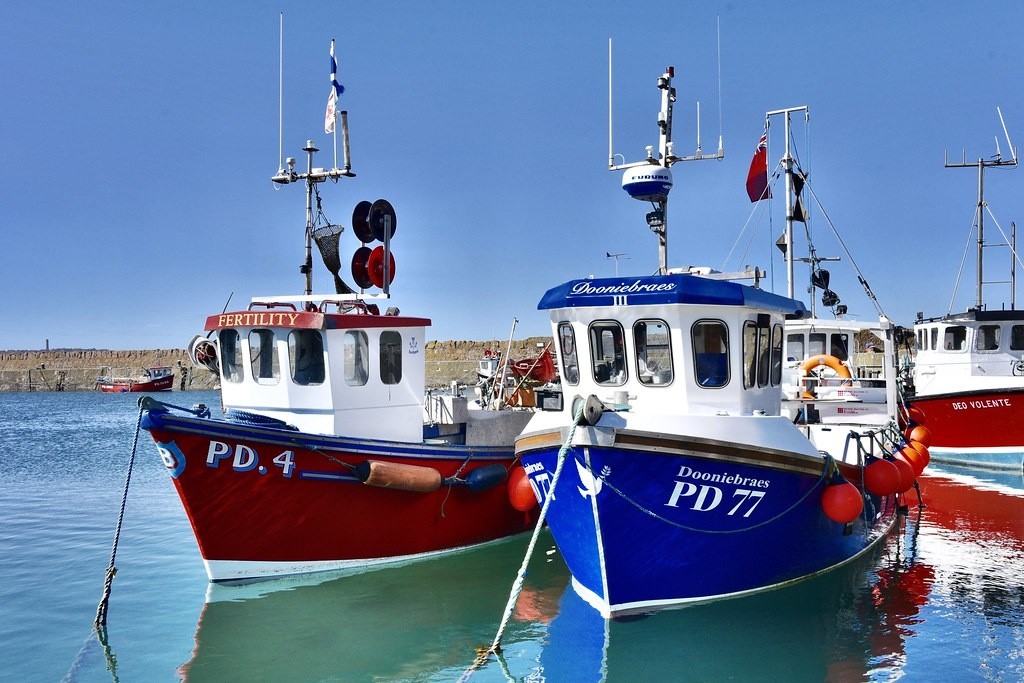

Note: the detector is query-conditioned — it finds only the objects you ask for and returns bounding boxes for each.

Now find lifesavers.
[796,354,852,399]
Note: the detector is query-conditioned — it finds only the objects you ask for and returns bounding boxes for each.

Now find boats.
[97,366,174,393]
[911,105,1023,469]
[138,13,931,616]
